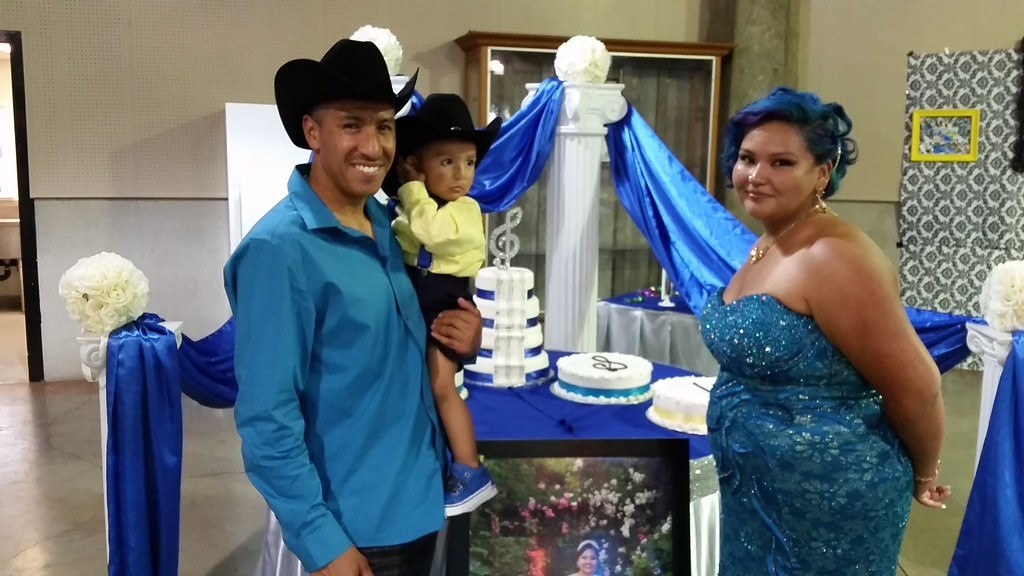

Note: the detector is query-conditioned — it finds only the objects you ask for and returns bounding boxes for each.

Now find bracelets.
[914,459,942,483]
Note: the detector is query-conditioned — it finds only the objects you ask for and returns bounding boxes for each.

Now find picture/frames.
[910,108,980,164]
[445,437,693,576]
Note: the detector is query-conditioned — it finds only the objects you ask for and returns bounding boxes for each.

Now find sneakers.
[444,461,499,517]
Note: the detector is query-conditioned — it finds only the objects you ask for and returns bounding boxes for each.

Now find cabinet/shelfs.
[453,29,738,297]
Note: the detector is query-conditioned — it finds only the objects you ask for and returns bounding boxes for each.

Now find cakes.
[555,353,653,400]
[650,377,718,426]
[464,267,550,382]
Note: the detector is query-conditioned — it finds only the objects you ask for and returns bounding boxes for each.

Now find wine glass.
[658,269,676,307]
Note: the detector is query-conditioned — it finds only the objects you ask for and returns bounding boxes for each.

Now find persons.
[381,93,499,518]
[700,86,952,576]
[567,538,601,576]
[222,37,483,576]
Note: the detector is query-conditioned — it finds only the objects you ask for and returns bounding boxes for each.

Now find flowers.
[347,24,404,78]
[552,35,614,85]
[58,250,150,336]
[978,260,1024,333]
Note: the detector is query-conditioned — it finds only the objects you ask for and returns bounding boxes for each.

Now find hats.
[381,93,502,203]
[274,38,419,150]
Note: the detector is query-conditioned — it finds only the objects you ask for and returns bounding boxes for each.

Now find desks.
[257,347,720,576]
[592,281,987,378]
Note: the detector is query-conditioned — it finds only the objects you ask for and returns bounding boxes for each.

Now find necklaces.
[749,202,826,264]
